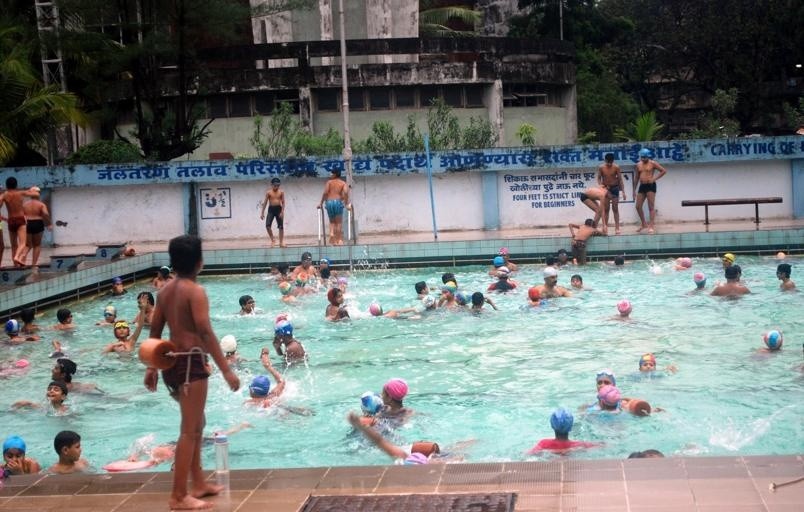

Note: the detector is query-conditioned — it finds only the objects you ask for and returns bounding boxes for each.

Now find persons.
[1,177,39,266]
[219,251,350,409]
[569,219,602,264]
[598,153,627,234]
[144,236,240,509]
[633,148,667,232]
[370,273,499,319]
[1,214,10,266]
[487,248,633,320]
[260,178,285,248]
[532,353,676,458]
[18,188,52,270]
[347,379,442,466]
[580,186,619,233]
[1,266,175,478]
[317,167,353,246]
[675,253,798,352]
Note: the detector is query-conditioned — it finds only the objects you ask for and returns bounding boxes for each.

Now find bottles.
[215,435,229,474]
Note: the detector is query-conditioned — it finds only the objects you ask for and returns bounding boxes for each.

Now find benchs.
[682,197,783,224]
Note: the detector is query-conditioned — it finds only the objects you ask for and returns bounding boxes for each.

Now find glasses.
[775,271,782,275]
[247,300,254,305]
[103,313,112,316]
[597,380,611,385]
[113,321,130,328]
